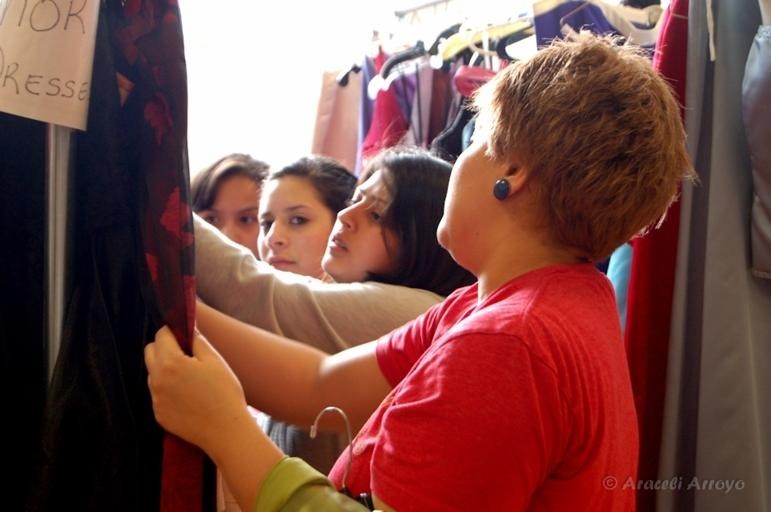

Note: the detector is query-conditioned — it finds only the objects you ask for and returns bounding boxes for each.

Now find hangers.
[371,14,540,81]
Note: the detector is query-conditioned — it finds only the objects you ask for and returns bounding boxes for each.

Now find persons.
[249,154,355,283]
[187,142,475,475]
[144,319,373,512]
[186,149,267,258]
[193,29,691,512]
